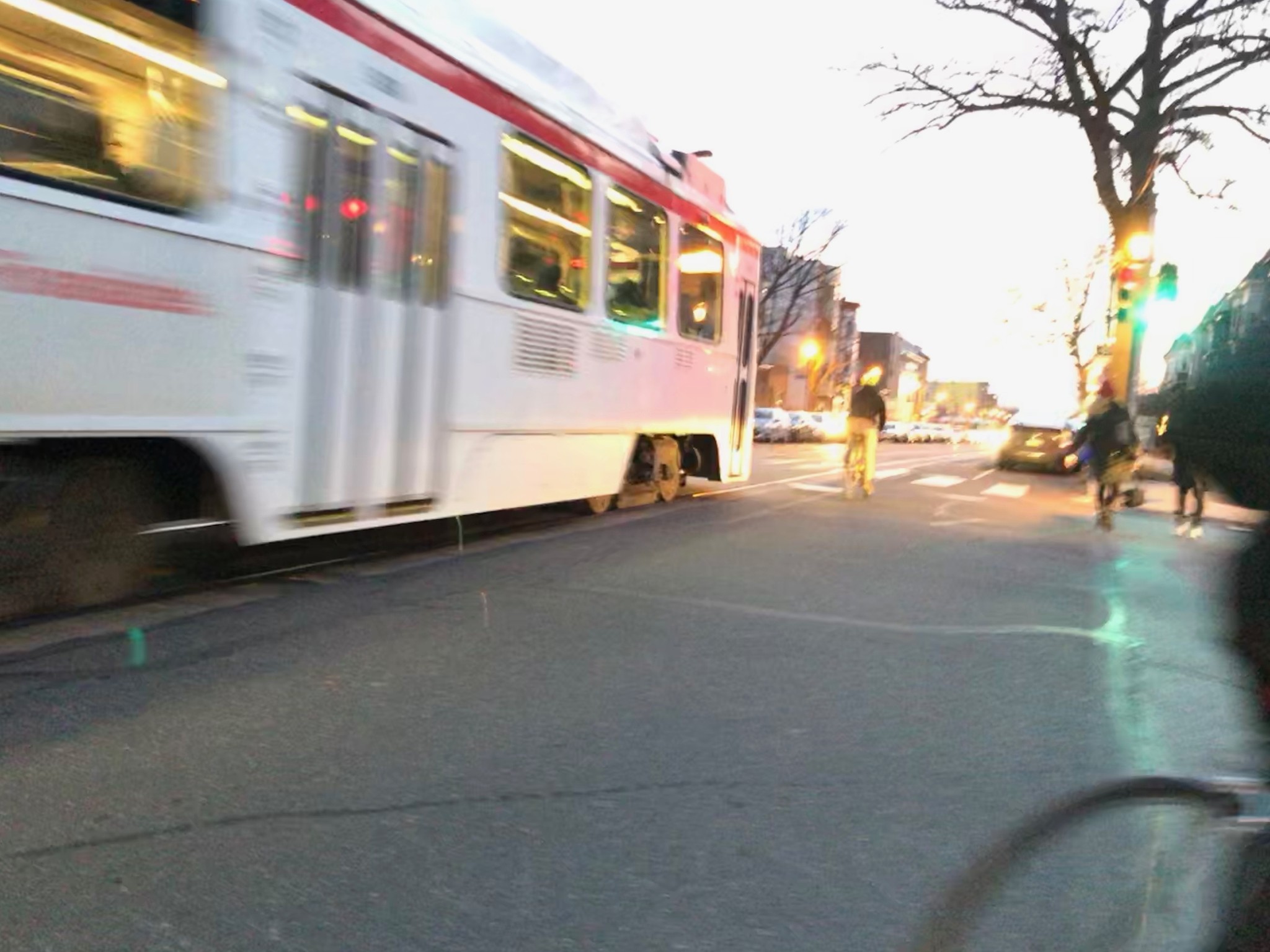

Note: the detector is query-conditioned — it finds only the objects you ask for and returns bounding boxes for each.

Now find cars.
[995,425,1083,474]
[753,406,971,444]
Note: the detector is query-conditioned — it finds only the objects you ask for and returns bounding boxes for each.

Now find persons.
[1064,380,1141,529]
[1161,381,1206,518]
[844,382,886,495]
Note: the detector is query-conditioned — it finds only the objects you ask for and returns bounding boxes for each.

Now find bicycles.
[842,424,886,500]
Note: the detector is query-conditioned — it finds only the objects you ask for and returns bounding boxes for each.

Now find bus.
[0,0,764,628]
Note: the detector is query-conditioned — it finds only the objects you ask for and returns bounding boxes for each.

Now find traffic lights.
[1116,229,1151,324]
